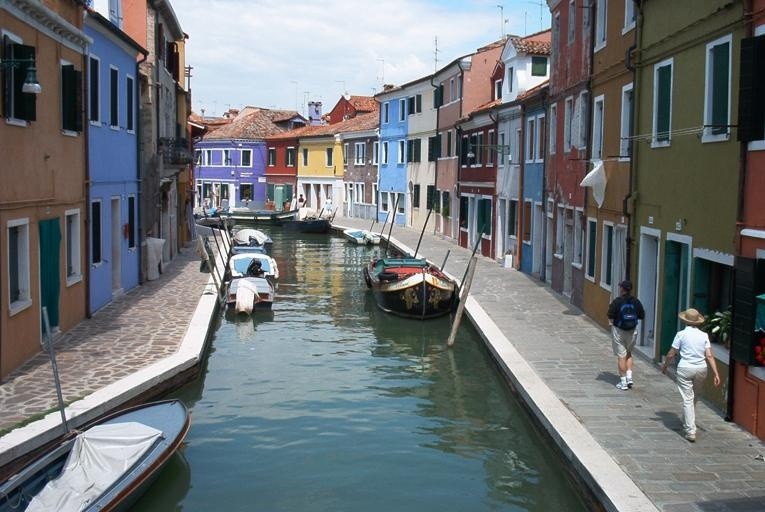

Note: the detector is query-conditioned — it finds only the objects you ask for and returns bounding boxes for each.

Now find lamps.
[0,55,43,95]
[466,139,511,159]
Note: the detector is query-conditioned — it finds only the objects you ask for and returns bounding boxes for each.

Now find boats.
[298,217,329,233]
[0,398,191,512]
[343,229,380,244]
[200,216,279,315]
[364,258,458,319]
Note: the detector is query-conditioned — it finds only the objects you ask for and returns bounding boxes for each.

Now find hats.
[678,308,705,325]
[618,280,633,290]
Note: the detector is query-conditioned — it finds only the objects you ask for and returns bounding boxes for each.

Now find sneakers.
[684,434,696,441]
[616,378,634,391]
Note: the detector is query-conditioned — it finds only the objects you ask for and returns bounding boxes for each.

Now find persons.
[325,195,335,218]
[659,308,721,441]
[606,281,645,390]
[298,193,304,204]
